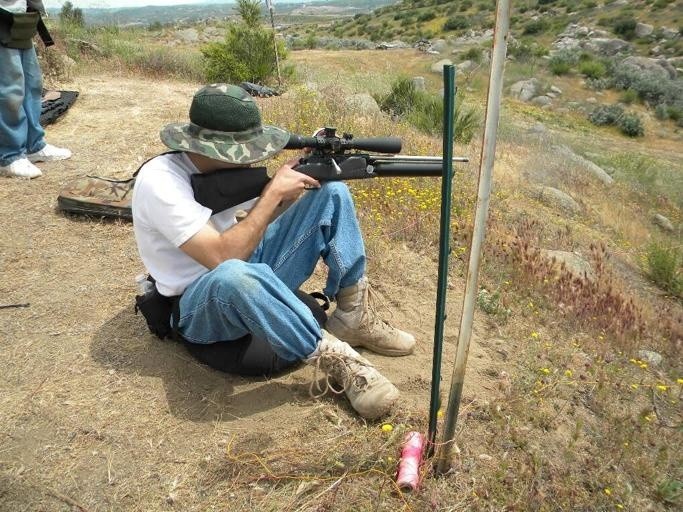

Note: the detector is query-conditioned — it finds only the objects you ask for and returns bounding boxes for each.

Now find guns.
[189,127,468,215]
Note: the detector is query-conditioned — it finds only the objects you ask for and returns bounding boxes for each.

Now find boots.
[304,277,415,420]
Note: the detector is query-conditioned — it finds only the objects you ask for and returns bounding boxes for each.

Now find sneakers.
[2,144,72,179]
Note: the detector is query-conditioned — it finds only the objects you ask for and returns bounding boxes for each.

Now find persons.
[131,79,419,422]
[0,1,74,180]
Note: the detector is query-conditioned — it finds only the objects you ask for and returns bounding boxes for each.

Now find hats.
[160,82,291,165]
